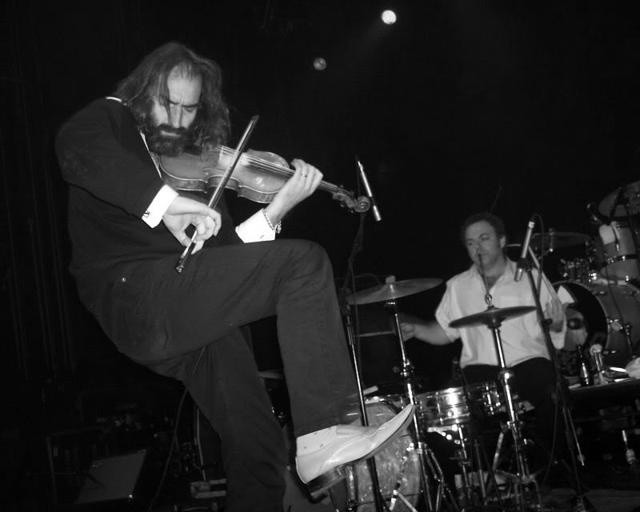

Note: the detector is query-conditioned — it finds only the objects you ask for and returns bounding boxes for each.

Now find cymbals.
[344,277,443,306]
[530,230,592,249]
[449,306,537,327]
[599,181,640,217]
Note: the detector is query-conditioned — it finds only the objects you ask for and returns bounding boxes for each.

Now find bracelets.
[262,209,284,236]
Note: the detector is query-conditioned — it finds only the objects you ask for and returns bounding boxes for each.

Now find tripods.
[347,296,549,512]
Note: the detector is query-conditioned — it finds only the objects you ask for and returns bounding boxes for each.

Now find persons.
[390,210,569,466]
[49,35,417,512]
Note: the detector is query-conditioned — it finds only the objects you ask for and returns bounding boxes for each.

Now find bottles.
[621,429,636,465]
[577,345,594,386]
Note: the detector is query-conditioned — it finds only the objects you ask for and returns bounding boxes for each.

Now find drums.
[413,379,505,432]
[543,274,640,376]
[587,217,640,280]
[277,391,437,512]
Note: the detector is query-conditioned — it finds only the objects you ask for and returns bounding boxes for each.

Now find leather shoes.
[294,403,416,499]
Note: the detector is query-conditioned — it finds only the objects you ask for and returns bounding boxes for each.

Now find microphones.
[515,221,535,281]
[356,154,382,223]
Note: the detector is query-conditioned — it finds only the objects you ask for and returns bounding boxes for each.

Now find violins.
[159,143,371,214]
